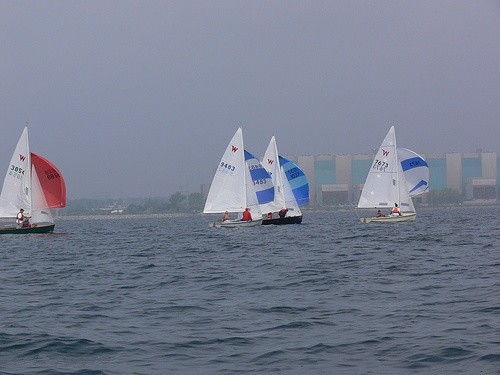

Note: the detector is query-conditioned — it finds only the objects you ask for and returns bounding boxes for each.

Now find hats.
[245,208,248,211]
[225,211,228,214]
[20,209,24,211]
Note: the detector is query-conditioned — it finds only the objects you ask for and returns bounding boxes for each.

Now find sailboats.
[0,120,67,235]
[259,135,312,225]
[201,127,274,227]
[354,123,431,223]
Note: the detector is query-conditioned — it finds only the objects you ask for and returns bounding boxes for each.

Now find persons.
[265,213,272,219]
[376,210,385,217]
[240,208,252,221]
[390,203,402,216]
[222,211,230,221]
[16,209,31,229]
[279,209,288,219]
[23,219,30,227]
